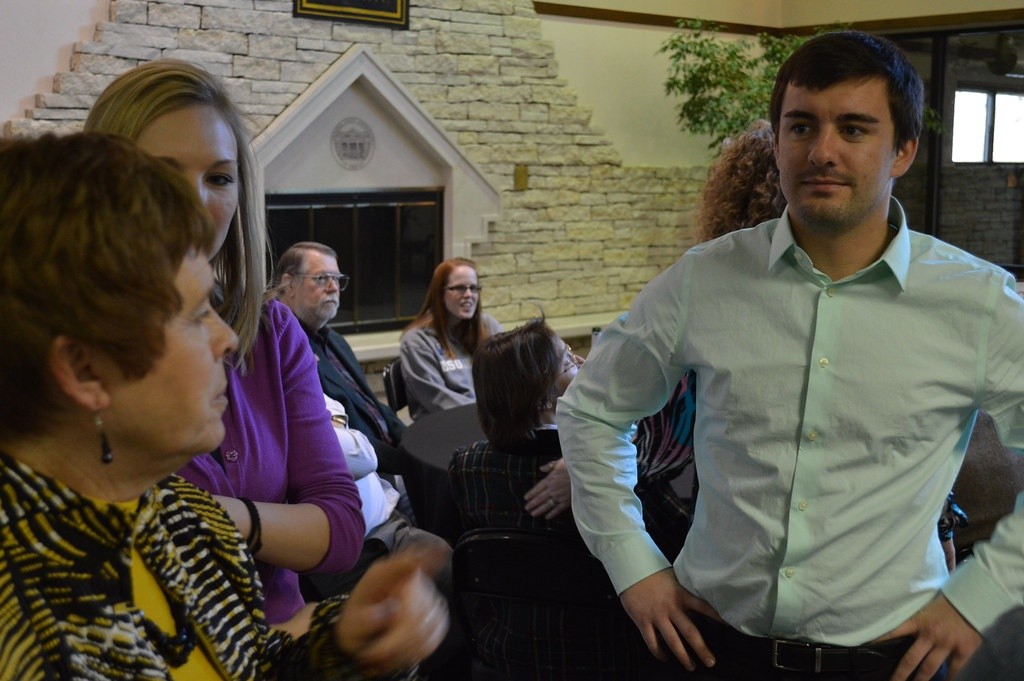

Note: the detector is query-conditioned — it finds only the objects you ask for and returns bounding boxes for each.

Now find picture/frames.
[292,0,410,31]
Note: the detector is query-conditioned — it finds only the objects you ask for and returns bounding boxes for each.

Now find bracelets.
[236,496,265,553]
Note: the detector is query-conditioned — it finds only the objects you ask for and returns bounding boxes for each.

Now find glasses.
[443,285,482,296]
[551,344,580,386]
[289,272,351,291]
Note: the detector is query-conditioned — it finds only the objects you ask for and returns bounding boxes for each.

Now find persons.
[523,365,697,521]
[522,117,967,573]
[82,56,368,620]
[553,27,1024,681]
[1,129,448,680]
[400,254,508,416]
[274,239,451,554]
[448,313,655,681]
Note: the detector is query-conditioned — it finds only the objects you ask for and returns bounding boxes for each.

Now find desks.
[399,403,489,542]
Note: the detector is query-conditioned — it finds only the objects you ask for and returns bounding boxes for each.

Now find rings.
[548,498,555,507]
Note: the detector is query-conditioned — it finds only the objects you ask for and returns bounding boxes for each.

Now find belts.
[691,611,915,674]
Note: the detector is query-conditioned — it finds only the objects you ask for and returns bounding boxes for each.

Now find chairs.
[382,357,415,424]
[450,527,628,666]
